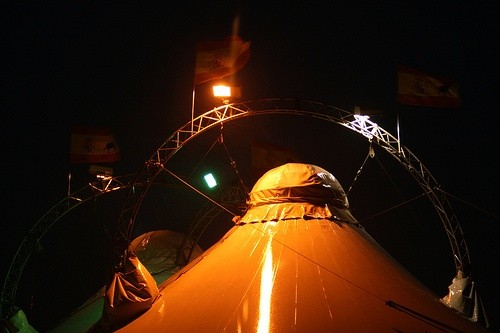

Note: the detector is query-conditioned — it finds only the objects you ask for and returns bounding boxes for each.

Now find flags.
[398,67,462,109]
[70,126,123,164]
[194,17,251,86]
